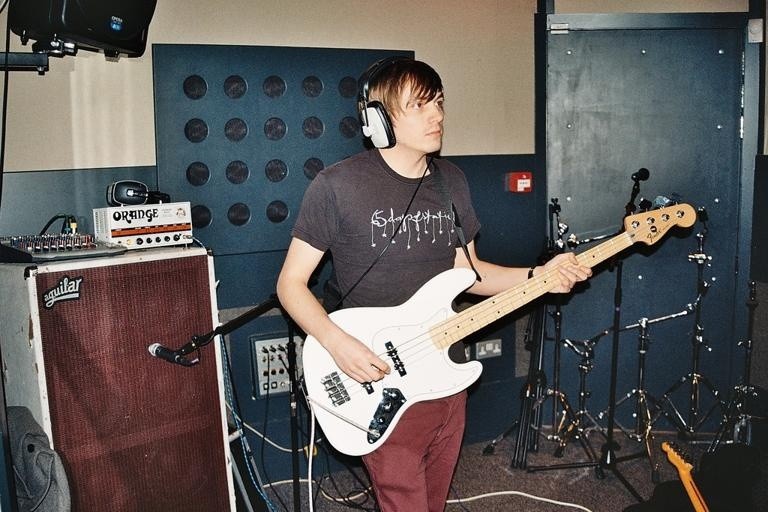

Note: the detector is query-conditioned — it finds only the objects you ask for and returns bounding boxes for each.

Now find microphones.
[148,343,193,367]
[631,168,649,181]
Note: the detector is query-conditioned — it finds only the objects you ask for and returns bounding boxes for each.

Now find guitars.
[302,192,697,457]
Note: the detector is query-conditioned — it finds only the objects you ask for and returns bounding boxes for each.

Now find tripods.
[483,180,768,503]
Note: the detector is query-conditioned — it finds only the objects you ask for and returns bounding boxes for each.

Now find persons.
[276,58,593,509]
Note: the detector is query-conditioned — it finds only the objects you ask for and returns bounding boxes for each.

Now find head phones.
[106,180,171,207]
[358,55,411,150]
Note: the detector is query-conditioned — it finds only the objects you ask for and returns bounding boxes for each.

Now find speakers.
[0,247,236,512]
[7,0,157,58]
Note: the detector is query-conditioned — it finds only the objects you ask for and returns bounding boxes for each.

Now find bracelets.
[529,263,535,280]
